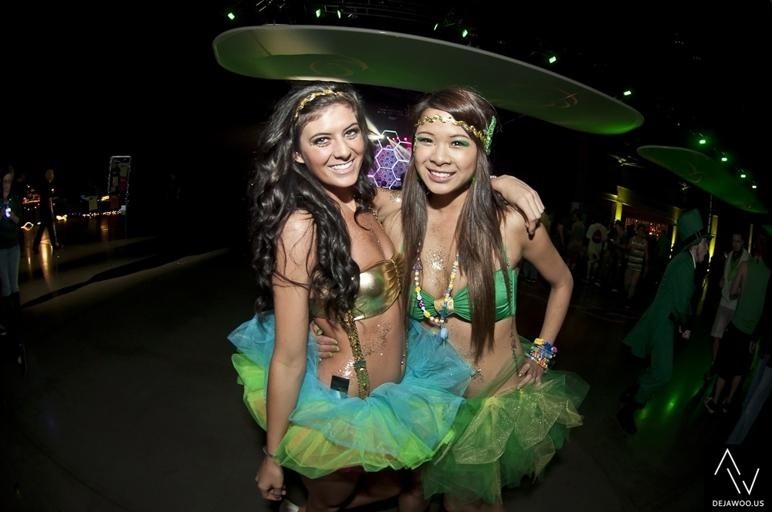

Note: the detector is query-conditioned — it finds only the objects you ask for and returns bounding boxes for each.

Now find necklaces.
[411,232,463,346]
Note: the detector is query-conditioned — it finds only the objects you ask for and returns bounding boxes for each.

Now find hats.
[674,209,713,256]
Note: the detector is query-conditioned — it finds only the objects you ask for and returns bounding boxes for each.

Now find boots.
[618,399,643,435]
[618,381,640,409]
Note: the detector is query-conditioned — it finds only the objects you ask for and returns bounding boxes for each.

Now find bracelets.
[262,445,281,462]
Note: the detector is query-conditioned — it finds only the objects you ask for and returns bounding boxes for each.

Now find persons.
[1,166,25,317]
[523,197,771,437]
[226,80,545,512]
[31,168,61,256]
[310,85,574,512]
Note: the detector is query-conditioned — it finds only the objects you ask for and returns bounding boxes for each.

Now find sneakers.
[720,401,729,412]
[705,398,717,413]
